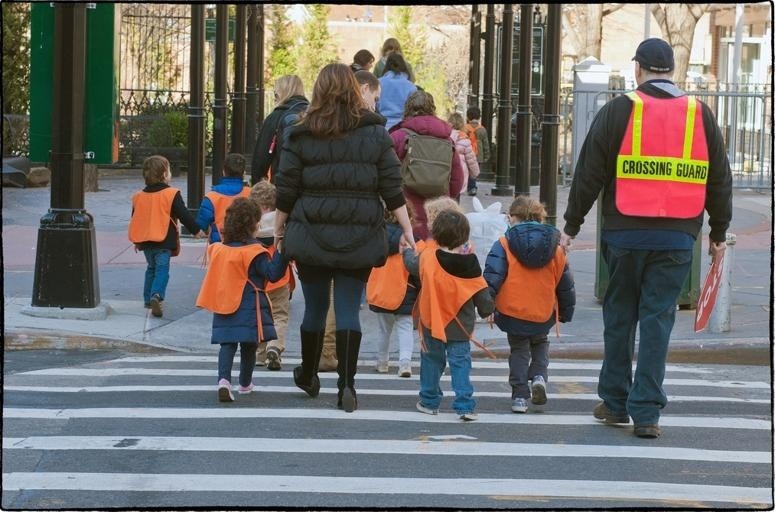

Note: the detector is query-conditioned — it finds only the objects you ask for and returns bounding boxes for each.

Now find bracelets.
[274,232,284,238]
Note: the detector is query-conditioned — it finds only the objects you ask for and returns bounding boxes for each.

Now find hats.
[631,37,675,74]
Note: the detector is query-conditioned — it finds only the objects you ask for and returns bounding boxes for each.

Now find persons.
[248,181,295,372]
[195,153,252,245]
[273,64,417,412]
[562,37,734,438]
[484,195,576,412]
[367,199,421,377]
[402,210,495,420]
[196,198,287,402]
[128,156,206,317]
[251,76,311,187]
[318,70,382,372]
[350,38,491,253]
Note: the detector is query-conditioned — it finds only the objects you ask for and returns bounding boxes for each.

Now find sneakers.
[633,422,662,439]
[459,410,478,421]
[266,345,282,371]
[531,375,548,405]
[397,365,412,377]
[238,381,256,395]
[317,350,339,372]
[511,397,529,414]
[416,400,439,415]
[149,294,163,318]
[592,399,631,425]
[375,362,389,373]
[216,378,235,402]
[144,300,163,309]
[255,346,267,366]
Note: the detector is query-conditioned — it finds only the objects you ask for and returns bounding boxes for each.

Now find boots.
[334,329,362,413]
[293,322,326,398]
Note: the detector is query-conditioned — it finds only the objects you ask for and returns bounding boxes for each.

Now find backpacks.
[398,126,459,199]
[272,112,303,158]
